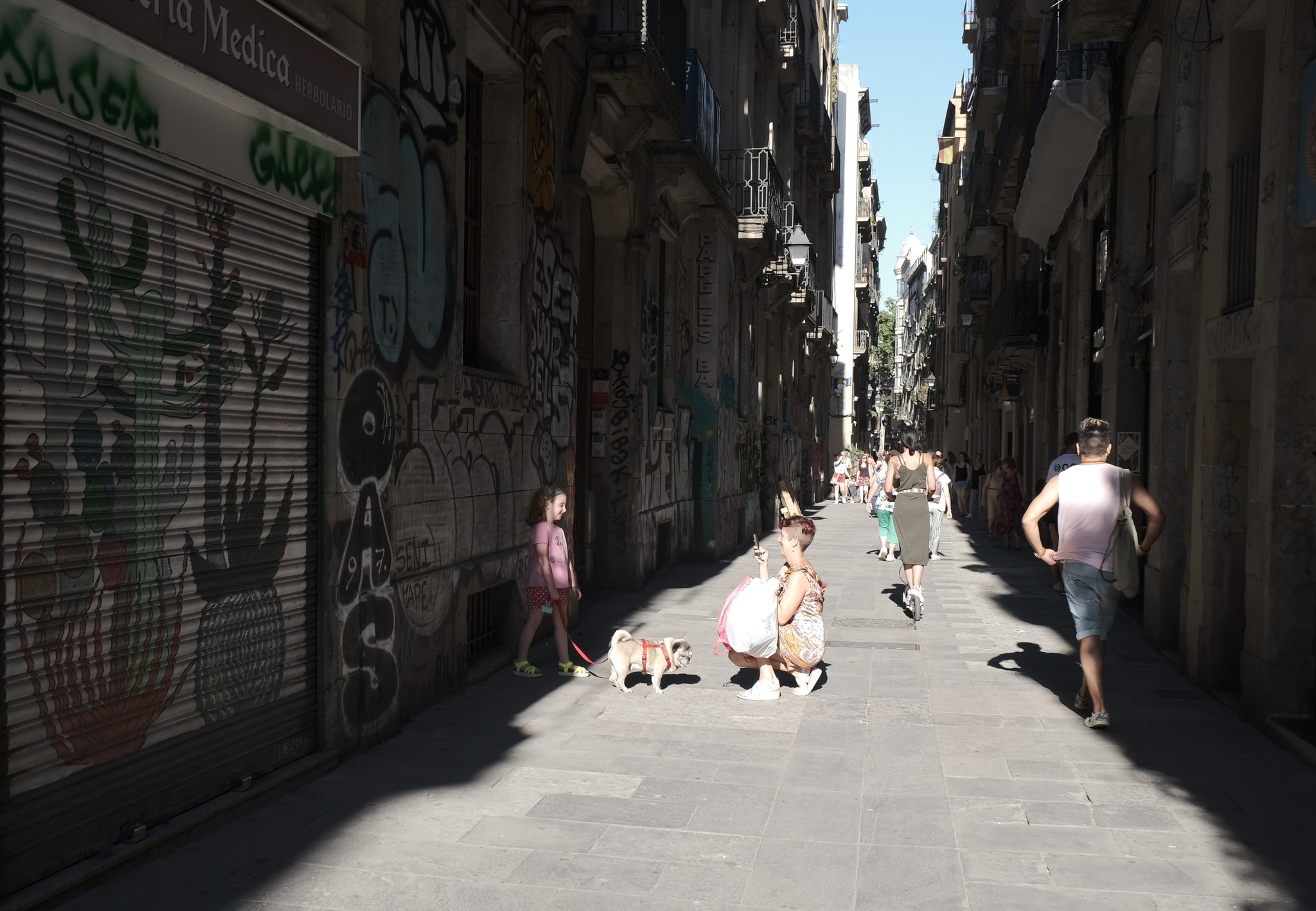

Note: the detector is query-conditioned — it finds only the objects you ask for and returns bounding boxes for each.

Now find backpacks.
[925,473,946,504]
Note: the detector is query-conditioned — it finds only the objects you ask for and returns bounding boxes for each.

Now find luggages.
[870,495,878,517]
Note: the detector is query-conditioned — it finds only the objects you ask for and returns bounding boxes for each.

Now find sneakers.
[878,546,888,561]
[834,497,867,504]
[886,553,896,562]
[906,584,925,603]
[931,554,941,561]
[1084,710,1110,727]
[1052,582,1064,592]
[1074,685,1093,710]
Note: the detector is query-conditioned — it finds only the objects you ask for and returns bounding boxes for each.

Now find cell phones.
[753,533,761,557]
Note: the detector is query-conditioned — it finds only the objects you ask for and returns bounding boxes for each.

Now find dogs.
[606,629,692,694]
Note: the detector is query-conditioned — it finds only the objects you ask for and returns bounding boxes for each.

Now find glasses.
[975,456,982,459]
[841,455,846,456]
[999,468,1003,471]
[862,458,867,459]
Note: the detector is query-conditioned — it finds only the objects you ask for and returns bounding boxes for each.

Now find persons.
[728,515,828,701]
[834,449,1026,562]
[512,484,589,678]
[1021,418,1166,728]
[1046,432,1082,596]
[884,426,936,621]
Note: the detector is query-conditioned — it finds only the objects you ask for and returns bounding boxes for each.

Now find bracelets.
[571,581,578,588]
[1033,547,1046,559]
[867,502,872,503]
[1139,544,1151,553]
[964,483,968,484]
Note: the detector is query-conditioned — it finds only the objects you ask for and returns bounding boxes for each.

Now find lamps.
[926,372,945,395]
[753,222,813,303]
[878,402,884,412]
[829,349,839,369]
[857,384,874,402]
[959,301,975,326]
[871,393,881,409]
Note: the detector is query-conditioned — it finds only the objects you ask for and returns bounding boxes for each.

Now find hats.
[935,451,942,458]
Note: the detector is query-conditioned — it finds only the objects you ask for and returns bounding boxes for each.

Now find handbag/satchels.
[830,477,838,484]
[725,577,779,659]
[844,468,851,478]
[1099,465,1139,598]
[892,456,901,489]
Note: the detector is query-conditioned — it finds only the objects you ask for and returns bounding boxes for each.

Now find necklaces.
[1080,460,1105,463]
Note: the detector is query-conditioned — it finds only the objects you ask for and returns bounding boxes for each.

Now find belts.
[898,488,926,494]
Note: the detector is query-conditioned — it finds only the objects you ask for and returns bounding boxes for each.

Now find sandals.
[957,509,1022,550]
[791,665,823,696]
[513,660,543,678]
[557,661,589,677]
[736,677,780,700]
